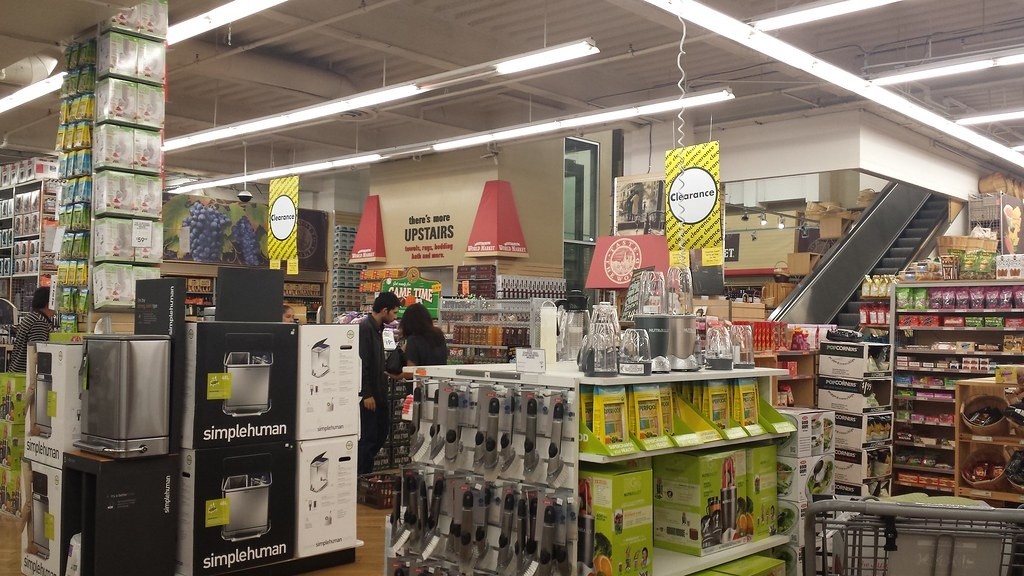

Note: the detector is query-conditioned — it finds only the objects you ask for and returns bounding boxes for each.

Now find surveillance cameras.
[236,191,254,203]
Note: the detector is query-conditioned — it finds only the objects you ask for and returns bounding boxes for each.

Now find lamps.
[162,1,735,194]
[746,0,905,33]
[759,213,768,226]
[751,234,757,241]
[954,107,1024,126]
[862,44,1024,87]
[779,217,785,229]
[741,210,749,221]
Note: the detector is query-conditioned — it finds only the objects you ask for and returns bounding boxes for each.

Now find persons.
[627,190,639,220]
[386,304,448,463]
[8,286,55,374]
[357,293,400,496]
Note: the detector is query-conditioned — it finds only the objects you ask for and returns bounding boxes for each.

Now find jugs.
[553,289,592,363]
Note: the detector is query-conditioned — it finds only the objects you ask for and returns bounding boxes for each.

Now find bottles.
[439,281,568,364]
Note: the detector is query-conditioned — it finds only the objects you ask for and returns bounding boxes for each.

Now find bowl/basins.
[776,544,797,573]
[778,501,799,535]
[777,413,799,449]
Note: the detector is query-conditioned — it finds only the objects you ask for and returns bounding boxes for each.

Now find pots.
[805,413,835,571]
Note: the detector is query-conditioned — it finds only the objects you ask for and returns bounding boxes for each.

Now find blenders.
[667,263,700,372]
[632,271,672,374]
[697,457,739,548]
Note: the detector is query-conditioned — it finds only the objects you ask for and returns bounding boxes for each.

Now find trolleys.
[801,497,1024,576]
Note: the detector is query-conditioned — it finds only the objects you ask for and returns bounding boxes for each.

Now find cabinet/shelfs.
[787,252,825,275]
[0,179,56,314]
[955,377,1024,503]
[184,291,324,325]
[858,279,1024,493]
[818,217,855,240]
[752,349,819,409]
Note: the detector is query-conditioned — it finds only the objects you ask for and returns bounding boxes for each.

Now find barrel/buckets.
[961,395,1024,494]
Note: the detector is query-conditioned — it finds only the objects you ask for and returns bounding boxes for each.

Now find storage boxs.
[457,265,497,299]
[0,157,59,277]
[92,0,169,310]
[398,341,895,576]
[187,278,211,292]
[578,443,786,576]
[896,341,998,489]
[329,225,360,319]
[176,320,359,576]
[0,333,87,576]
[282,282,322,296]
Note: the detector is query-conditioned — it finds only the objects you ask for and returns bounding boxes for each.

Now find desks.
[60,451,181,576]
